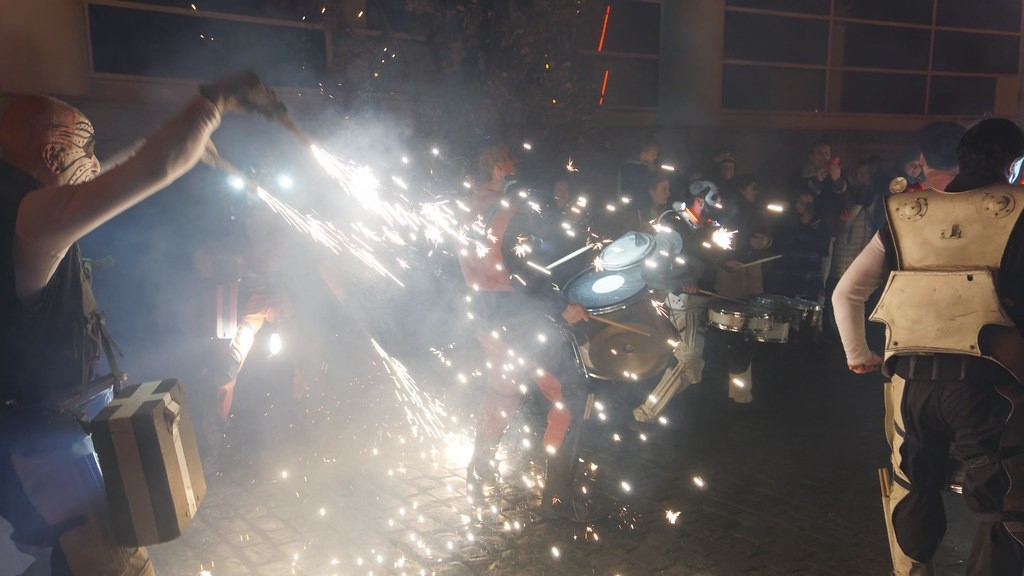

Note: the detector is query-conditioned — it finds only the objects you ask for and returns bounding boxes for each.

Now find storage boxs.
[90,378,207,549]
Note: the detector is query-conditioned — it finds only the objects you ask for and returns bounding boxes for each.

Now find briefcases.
[90,377,206,545]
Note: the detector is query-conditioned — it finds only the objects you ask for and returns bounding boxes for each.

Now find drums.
[599,230,657,272]
[560,264,677,383]
[745,312,792,344]
[707,303,774,332]
[751,293,823,327]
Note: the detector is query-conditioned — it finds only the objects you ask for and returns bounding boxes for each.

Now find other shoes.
[630,422,671,439]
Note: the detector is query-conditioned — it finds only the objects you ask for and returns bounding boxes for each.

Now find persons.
[891,149,924,187]
[904,120,968,193]
[0,74,234,576]
[626,179,770,436]
[444,138,606,522]
[533,153,888,362]
[798,138,849,195]
[831,118,1024,576]
[613,138,659,220]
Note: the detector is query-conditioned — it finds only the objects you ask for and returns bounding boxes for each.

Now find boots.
[465,456,501,525]
[541,454,610,522]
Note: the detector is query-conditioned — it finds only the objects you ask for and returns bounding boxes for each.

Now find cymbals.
[641,254,706,290]
[588,321,671,382]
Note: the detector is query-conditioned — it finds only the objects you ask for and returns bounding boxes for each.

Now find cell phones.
[805,202,814,215]
[829,156,840,166]
